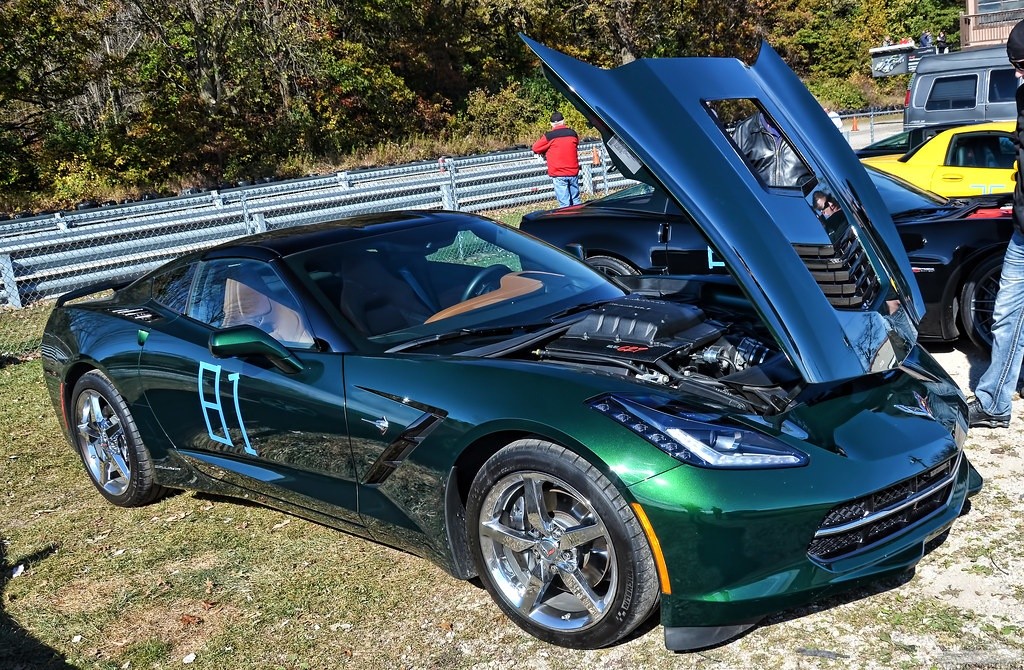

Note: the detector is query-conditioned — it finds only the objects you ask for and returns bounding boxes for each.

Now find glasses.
[1009,60,1024,71]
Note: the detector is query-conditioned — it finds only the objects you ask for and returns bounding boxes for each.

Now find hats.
[550,113,564,122]
[1006,19,1024,60]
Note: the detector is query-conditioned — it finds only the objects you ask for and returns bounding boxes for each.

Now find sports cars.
[858,121,1018,197]
[40,32,983,652]
[518,161,1013,356]
[875,54,905,74]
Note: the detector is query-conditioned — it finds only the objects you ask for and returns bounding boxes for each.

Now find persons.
[730,110,813,187]
[964,18,1024,429]
[936,31,946,53]
[823,103,844,134]
[813,191,841,216]
[532,113,581,208]
[881,30,933,47]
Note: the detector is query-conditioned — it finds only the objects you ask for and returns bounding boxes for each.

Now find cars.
[855,120,993,159]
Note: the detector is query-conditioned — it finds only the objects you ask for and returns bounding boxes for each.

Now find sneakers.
[966,395,1011,428]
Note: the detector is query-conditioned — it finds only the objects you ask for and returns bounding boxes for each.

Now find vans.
[903,44,1024,133]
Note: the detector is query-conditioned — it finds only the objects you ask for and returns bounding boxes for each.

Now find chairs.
[335,246,433,341]
[218,268,314,342]
[956,145,978,166]
[983,146,1000,168]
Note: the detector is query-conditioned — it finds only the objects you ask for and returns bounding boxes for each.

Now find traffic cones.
[592,147,601,165]
[851,116,859,131]
